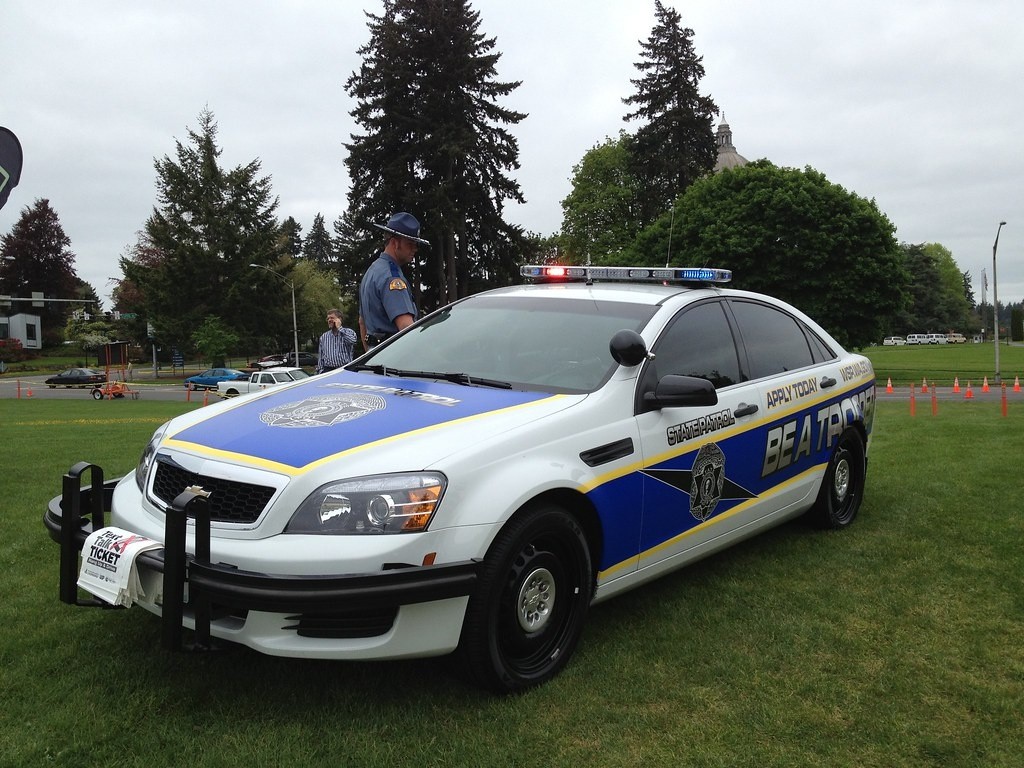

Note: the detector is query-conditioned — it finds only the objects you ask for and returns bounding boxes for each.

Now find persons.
[359,212,431,351]
[316,309,357,373]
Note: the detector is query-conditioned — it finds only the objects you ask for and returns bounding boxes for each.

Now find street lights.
[248,263,300,368]
[992,221,1008,385]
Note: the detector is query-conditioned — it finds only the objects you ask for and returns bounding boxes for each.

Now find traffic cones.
[962,381,975,398]
[885,376,894,394]
[951,376,962,393]
[920,376,930,393]
[1012,376,1022,392]
[979,375,993,393]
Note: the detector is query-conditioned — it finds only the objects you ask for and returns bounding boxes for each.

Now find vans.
[946,334,967,344]
[926,334,949,345]
[906,334,932,345]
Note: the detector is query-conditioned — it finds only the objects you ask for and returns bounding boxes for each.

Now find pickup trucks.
[216,366,310,400]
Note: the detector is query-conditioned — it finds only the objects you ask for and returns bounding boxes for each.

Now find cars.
[184,368,250,391]
[257,355,286,362]
[283,352,319,366]
[40,262,877,694]
[44,367,106,389]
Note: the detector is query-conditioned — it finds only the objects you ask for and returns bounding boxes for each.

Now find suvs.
[883,336,907,346]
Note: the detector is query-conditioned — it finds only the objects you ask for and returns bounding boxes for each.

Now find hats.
[373,212,429,245]
